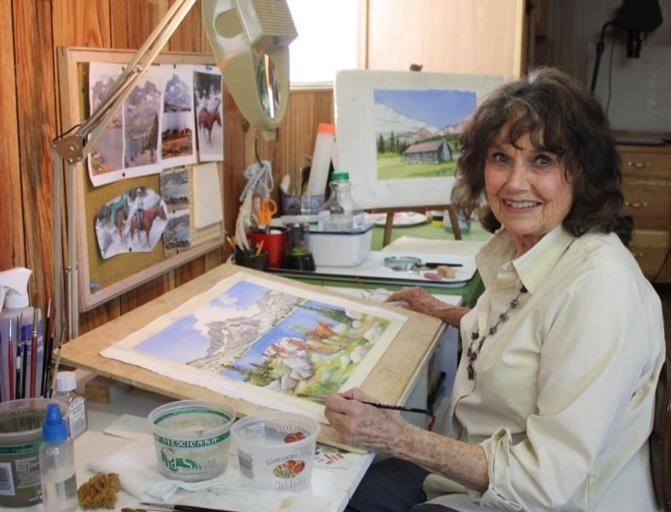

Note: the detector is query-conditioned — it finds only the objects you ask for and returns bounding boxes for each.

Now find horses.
[127,205,168,245]
[198,108,222,143]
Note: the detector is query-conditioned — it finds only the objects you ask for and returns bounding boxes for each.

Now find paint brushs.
[226,234,265,256]
[416,263,463,268]
[297,393,433,415]
[0,292,66,402]
[120,503,237,512]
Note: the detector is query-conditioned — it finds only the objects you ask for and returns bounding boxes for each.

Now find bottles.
[54,371,88,441]
[38,404,79,512]
[316,170,365,235]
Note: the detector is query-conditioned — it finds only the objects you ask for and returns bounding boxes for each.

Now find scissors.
[252,192,277,235]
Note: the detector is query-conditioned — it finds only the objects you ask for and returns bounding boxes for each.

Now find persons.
[324,64,669,512]
[200,88,209,112]
[135,189,147,228]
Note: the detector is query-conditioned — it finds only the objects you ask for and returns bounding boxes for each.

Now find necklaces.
[467,282,526,383]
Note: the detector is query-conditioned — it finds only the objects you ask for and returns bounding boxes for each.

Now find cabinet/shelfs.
[602,127,671,289]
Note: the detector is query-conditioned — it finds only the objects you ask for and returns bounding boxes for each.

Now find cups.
[234,227,287,270]
[147,398,237,487]
[0,398,70,508]
[230,408,320,494]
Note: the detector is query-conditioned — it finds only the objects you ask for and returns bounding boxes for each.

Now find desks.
[47,292,464,512]
